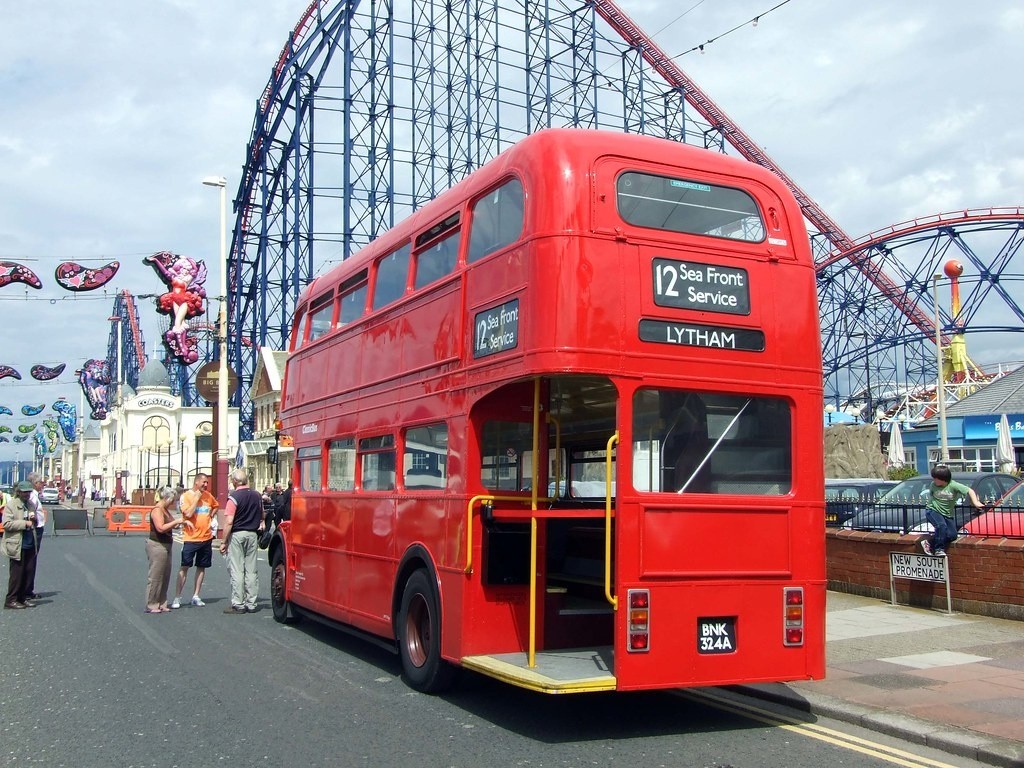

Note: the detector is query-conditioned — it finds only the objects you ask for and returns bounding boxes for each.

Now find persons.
[219,470,263,614]
[921,466,985,556]
[172,473,219,608]
[211,516,217,539]
[65,485,87,499]
[261,480,292,532]
[0,472,45,608]
[144,487,195,613]
[121,486,126,504]
[91,483,107,506]
[112,487,116,505]
[175,482,185,515]
[155,485,171,503]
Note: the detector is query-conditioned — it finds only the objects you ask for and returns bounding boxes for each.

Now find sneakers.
[935,549,946,557]
[920,539,933,555]
[171,598,181,608]
[191,596,206,607]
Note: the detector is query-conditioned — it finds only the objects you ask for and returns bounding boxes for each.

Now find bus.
[266,126,832,706]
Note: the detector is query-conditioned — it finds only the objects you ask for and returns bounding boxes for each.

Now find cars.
[824,477,902,528]
[840,472,1022,534]
[42,487,59,504]
[956,481,1024,541]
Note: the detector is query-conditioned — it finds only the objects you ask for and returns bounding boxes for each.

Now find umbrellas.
[997,414,1016,474]
[889,421,905,470]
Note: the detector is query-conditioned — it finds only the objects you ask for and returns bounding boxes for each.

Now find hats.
[18,481,33,492]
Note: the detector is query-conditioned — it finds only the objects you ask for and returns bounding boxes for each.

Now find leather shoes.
[4,601,26,609]
[22,600,36,607]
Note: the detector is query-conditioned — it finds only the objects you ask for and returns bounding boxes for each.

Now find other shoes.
[247,607,257,613]
[26,596,31,600]
[158,606,169,612]
[223,608,245,614]
[144,608,161,613]
[30,594,41,599]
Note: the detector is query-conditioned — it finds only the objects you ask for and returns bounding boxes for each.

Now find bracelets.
[221,541,227,544]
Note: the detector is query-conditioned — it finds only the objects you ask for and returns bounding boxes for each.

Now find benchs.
[489,521,615,614]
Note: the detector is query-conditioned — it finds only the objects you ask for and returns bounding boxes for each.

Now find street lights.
[932,273,950,462]
[203,174,232,540]
[30,368,86,496]
[107,316,123,501]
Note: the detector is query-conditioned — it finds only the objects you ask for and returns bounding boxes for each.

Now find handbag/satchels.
[256,530,272,550]
